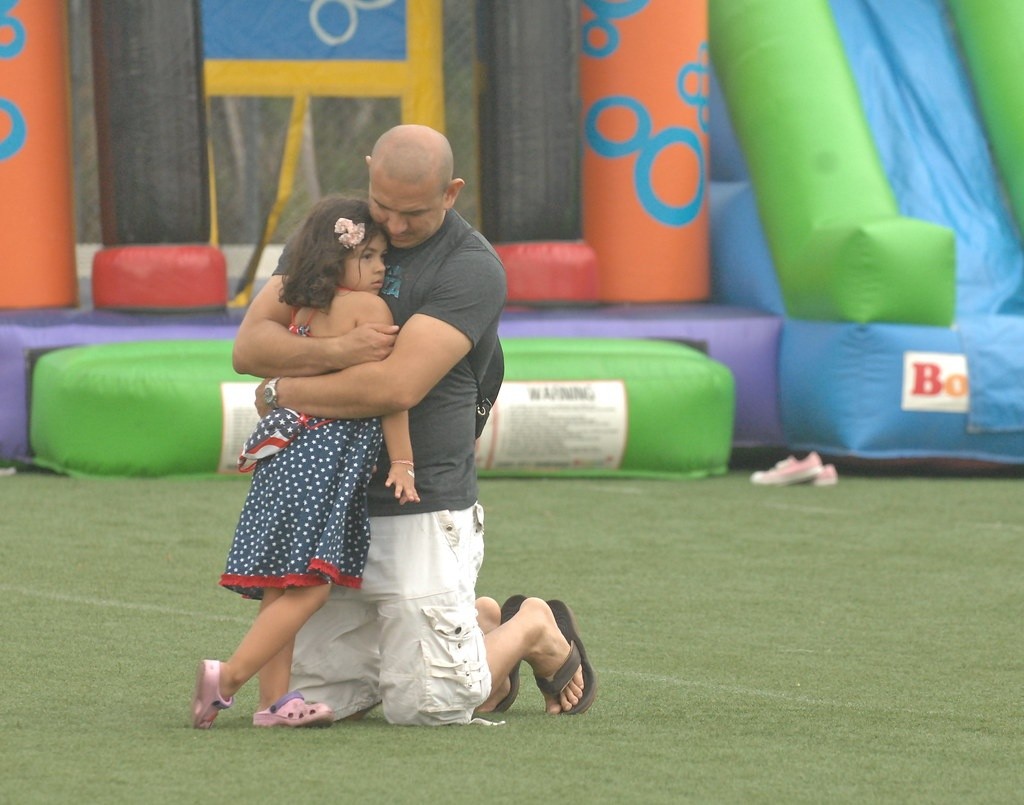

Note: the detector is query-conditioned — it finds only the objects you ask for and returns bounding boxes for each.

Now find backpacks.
[440,226,505,439]
[237,308,337,473]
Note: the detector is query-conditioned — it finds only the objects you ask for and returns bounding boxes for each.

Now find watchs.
[264,377,283,411]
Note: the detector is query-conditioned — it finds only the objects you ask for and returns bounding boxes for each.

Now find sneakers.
[751,451,838,486]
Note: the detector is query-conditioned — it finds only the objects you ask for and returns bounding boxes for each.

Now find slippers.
[253,691,334,728]
[492,594,530,712]
[534,599,597,715]
[190,660,233,729]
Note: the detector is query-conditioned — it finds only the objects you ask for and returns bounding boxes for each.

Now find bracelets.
[390,459,414,467]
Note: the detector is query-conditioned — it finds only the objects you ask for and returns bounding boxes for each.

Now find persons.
[186,196,422,726]
[233,121,597,725]
